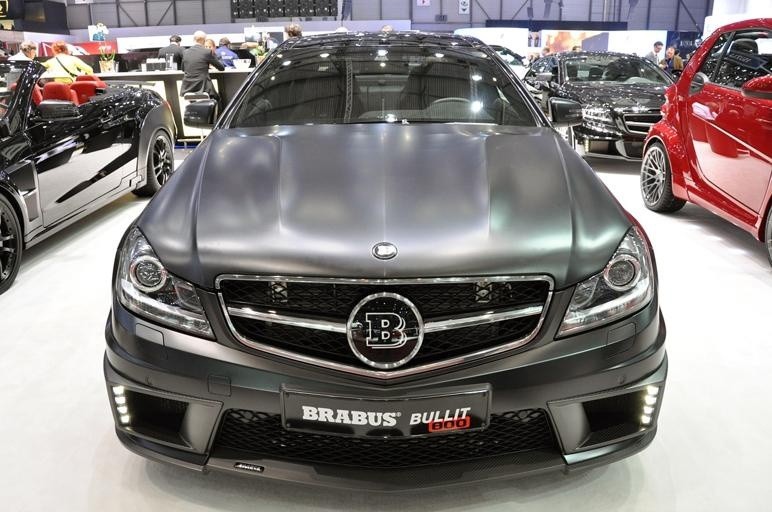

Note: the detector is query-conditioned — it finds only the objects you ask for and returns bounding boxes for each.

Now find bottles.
[257,32,271,56]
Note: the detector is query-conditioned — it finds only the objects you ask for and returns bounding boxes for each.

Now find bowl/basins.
[233,59,252,68]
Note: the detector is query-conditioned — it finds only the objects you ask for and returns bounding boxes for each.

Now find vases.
[99,60,116,73]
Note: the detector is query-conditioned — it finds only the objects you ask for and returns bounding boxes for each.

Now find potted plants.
[255,31,269,68]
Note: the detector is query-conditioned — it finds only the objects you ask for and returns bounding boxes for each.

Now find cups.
[164,52,174,71]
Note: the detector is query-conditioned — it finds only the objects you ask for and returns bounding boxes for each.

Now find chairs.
[75,74,108,89]
[70,80,99,107]
[184,92,218,127]
[588,68,603,81]
[42,82,72,104]
[721,38,772,87]
[396,62,501,119]
[9,83,42,110]
[567,64,583,82]
[230,58,365,128]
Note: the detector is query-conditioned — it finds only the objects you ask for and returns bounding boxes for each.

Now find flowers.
[92,23,115,61]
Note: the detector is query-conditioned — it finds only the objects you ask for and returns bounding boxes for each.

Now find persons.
[204,39,219,68]
[158,35,185,70]
[181,31,226,124]
[660,47,674,75]
[215,37,239,66]
[672,49,682,69]
[43,41,93,79]
[7,41,36,61]
[645,41,663,66]
